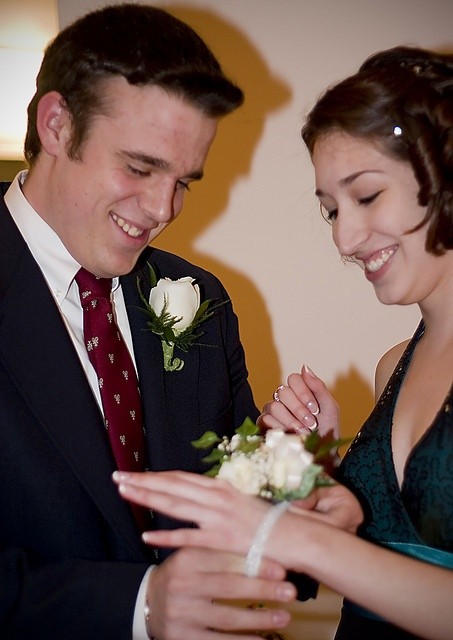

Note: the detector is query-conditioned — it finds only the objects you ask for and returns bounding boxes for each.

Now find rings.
[274,385,290,402]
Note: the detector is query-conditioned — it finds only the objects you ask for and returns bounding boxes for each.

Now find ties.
[74,268,161,553]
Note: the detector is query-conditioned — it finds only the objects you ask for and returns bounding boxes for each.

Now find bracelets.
[243,501,292,577]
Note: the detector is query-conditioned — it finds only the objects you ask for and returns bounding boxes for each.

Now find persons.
[2,3,364,638]
[112,46,452,639]
